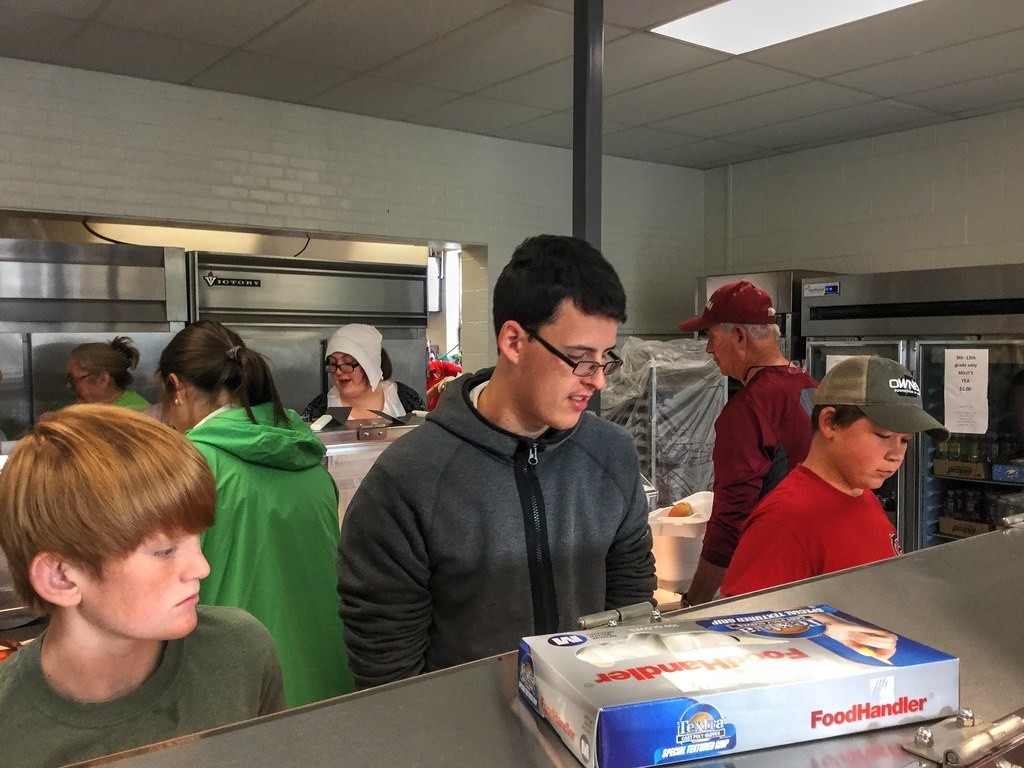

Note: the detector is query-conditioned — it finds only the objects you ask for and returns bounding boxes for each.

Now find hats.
[813,354,951,442]
[677,281,776,332]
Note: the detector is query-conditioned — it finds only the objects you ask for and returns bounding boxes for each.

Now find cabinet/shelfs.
[804,339,907,555]
[910,339,1024,552]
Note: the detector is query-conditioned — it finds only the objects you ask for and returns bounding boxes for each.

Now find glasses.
[526,328,623,377]
[66,374,94,385]
[324,361,359,373]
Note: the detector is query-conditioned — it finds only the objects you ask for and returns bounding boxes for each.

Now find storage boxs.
[933,458,991,480]
[939,515,994,538]
[992,461,1024,484]
[517,601,963,767]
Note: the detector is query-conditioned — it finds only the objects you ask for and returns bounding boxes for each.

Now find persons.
[0,404,286,768]
[301,323,426,430]
[677,281,820,609]
[65,336,152,410]
[426,338,462,412]
[336,235,658,691]
[156,320,356,710]
[718,357,951,599]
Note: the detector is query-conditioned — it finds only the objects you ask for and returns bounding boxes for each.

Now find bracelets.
[681,592,693,608]
